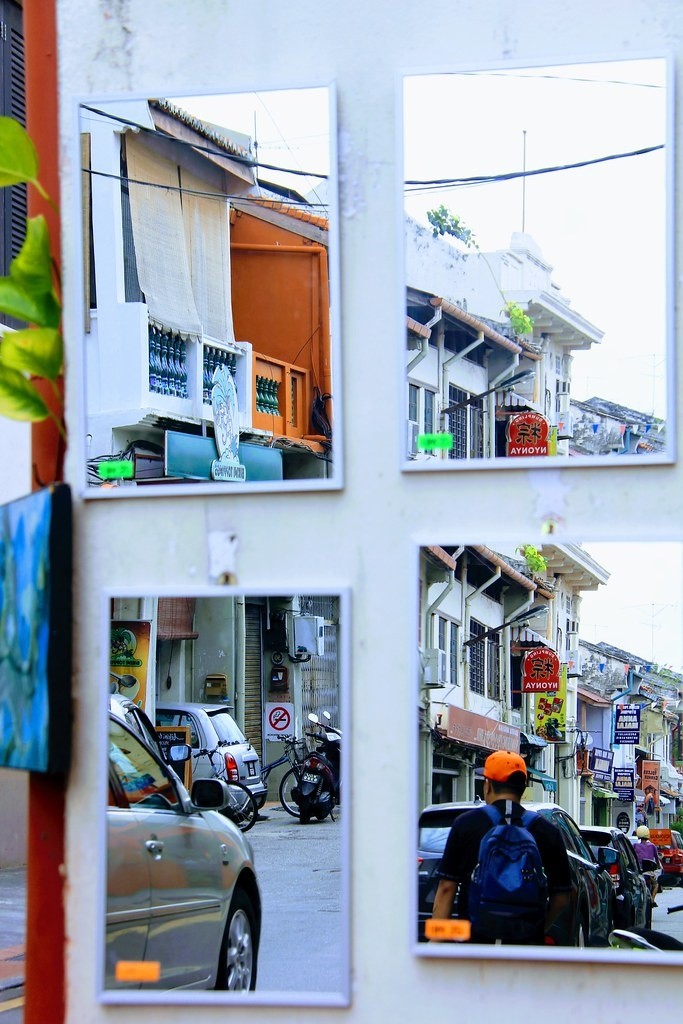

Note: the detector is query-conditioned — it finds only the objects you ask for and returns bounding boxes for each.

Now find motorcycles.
[291,709,341,825]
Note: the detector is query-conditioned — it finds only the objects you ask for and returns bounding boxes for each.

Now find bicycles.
[259,733,310,819]
[194,739,258,834]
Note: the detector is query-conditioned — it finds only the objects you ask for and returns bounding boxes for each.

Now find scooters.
[609,905,683,951]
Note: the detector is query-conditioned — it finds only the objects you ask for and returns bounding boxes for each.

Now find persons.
[631,824,661,907]
[430,748,572,946]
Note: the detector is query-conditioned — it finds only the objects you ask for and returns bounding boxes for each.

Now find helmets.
[636,825,650,838]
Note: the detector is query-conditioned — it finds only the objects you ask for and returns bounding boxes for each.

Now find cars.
[577,826,652,926]
[650,828,683,878]
[109,691,268,991]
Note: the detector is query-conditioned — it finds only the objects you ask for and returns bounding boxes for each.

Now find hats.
[475,751,527,782]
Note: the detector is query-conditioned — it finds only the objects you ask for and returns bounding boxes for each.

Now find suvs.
[418,803,620,947]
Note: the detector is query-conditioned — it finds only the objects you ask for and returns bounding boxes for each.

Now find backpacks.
[469,804,549,925]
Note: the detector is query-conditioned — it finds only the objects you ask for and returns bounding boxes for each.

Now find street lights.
[439,367,537,461]
[461,601,548,801]
